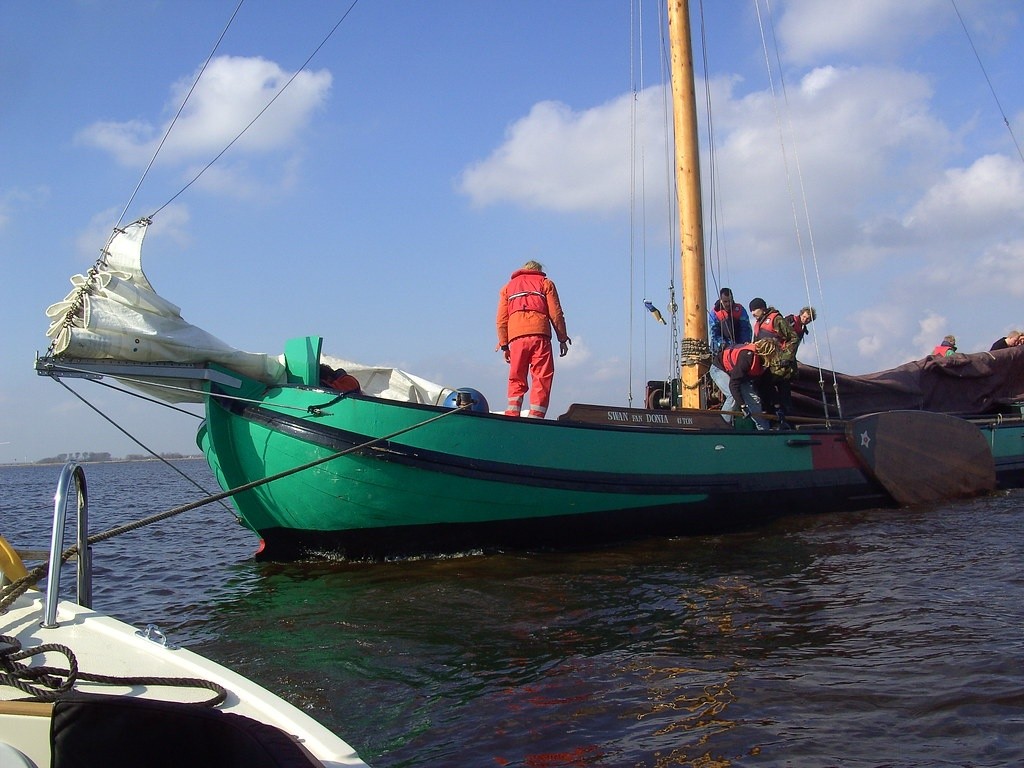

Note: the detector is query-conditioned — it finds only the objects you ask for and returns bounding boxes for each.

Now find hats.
[749,297,766,310]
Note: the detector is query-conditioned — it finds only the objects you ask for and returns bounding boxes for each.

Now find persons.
[319,363,362,394]
[990,331,1024,351]
[932,335,957,357]
[710,286,817,429]
[497,260,568,419]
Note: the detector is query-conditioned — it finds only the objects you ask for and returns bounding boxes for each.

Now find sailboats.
[31,1,1023,568]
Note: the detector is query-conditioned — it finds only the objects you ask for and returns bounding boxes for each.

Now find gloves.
[776,408,784,424]
[741,405,750,420]
